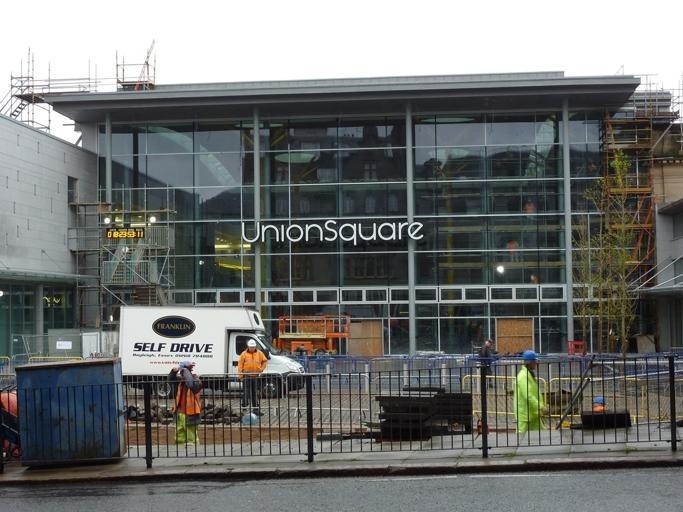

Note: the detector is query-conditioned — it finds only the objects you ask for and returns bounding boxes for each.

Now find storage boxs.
[344,321,385,356]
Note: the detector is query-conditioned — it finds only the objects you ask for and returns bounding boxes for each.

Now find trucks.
[118,304,306,399]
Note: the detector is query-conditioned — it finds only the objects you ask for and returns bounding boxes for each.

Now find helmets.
[247,339,256,346]
[522,350,538,360]
[592,397,606,405]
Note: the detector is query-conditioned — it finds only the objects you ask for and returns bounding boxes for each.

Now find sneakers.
[252,409,264,416]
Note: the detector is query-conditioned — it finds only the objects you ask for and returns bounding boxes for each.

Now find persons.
[481,339,497,390]
[530,273,539,284]
[296,343,305,352]
[168,361,202,445]
[514,349,551,435]
[591,396,609,412]
[506,239,522,263]
[314,343,328,355]
[238,338,264,417]
[524,198,536,222]
